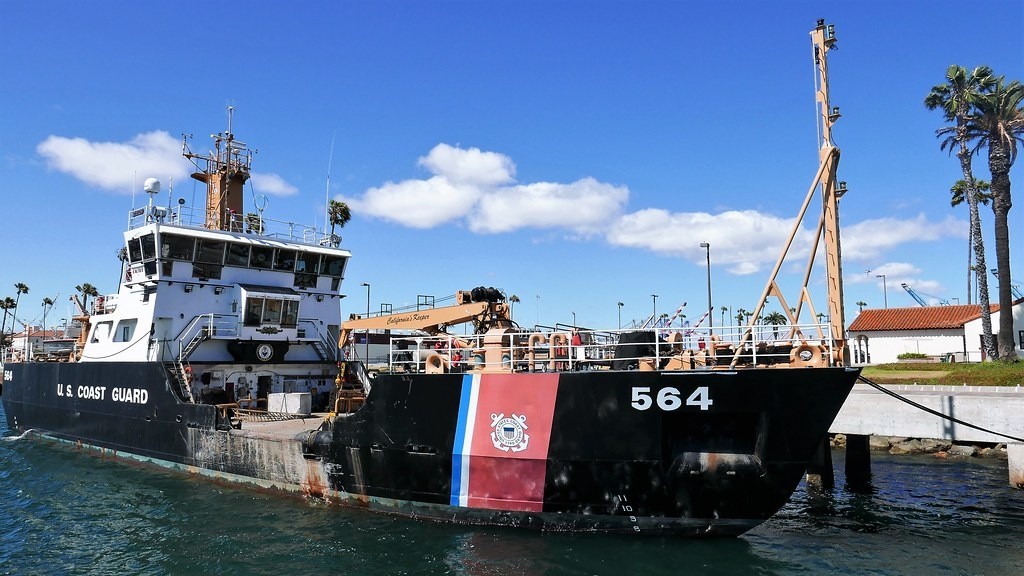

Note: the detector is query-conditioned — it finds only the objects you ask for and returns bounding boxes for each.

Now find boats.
[1,18,864,542]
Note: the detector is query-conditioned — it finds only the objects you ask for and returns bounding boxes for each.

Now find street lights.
[618,302,624,329]
[700,243,713,336]
[651,295,658,323]
[361,283,370,371]
[952,298,959,305]
[876,275,887,308]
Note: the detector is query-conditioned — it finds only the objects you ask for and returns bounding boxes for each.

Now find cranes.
[991,269,1024,300]
[901,283,950,307]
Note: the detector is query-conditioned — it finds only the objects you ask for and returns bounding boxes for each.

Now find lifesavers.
[445,339,461,366]
